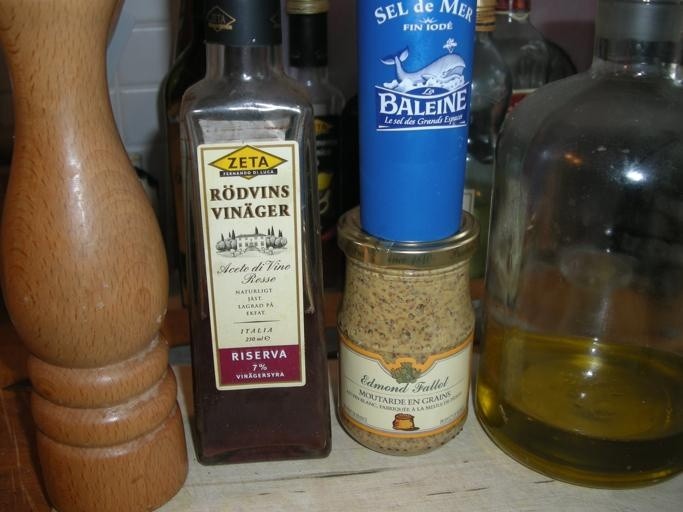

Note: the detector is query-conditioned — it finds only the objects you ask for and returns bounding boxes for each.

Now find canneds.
[335,207,478,456]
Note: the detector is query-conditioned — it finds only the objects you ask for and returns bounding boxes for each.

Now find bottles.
[351,2,477,239]
[149,4,329,468]
[269,2,355,215]
[481,5,683,487]
[468,3,561,190]
